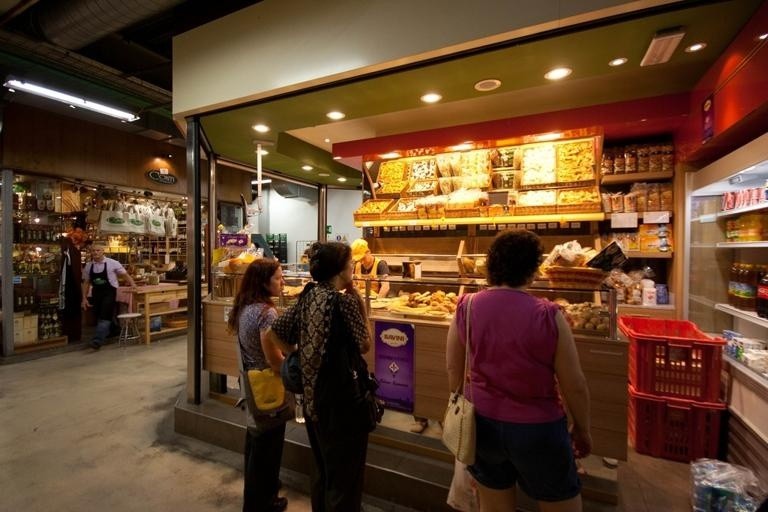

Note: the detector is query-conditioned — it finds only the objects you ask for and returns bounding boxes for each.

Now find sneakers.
[272,497,288,512]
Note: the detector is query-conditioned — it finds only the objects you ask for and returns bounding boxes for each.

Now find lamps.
[3,74,140,124]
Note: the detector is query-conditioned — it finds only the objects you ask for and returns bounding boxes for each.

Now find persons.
[446,227,592,510]
[349,239,390,299]
[262,242,373,512]
[224,256,297,512]
[80,246,144,350]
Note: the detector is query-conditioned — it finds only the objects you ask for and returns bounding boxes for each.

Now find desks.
[123,283,208,346]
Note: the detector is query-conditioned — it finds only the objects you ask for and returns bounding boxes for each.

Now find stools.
[117,313,142,346]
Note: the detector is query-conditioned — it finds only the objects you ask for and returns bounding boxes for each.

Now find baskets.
[543,265,610,290]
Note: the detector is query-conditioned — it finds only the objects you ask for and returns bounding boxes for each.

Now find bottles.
[728,261,768,318]
[725,213,768,242]
[601,145,674,175]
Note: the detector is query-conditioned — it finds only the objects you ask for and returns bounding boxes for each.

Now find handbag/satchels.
[243,367,296,425]
[315,309,386,434]
[440,392,477,465]
[100,201,177,237]
[283,349,304,395]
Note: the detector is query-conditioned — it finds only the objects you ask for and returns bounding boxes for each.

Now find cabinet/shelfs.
[600,169,675,310]
[714,203,768,391]
[13,207,68,354]
[203,274,629,465]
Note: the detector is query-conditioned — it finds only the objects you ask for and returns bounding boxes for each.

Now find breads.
[553,298,610,331]
[405,290,459,319]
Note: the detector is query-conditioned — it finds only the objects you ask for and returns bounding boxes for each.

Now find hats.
[350,239,368,261]
